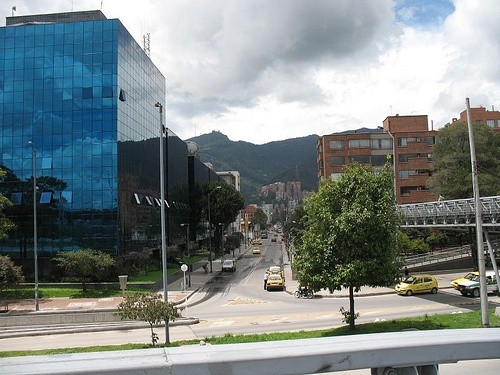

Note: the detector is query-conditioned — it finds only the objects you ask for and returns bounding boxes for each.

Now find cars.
[252,246,261,254]
[266,274,283,291]
[395,274,439,296]
[251,223,282,244]
[452,272,478,291]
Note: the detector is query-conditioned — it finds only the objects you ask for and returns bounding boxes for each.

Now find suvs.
[222,260,236,271]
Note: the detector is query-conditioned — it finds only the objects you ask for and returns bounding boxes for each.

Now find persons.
[405,267,408,280]
[263,271,268,289]
[203,261,208,273]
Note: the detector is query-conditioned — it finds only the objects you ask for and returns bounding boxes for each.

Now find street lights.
[377,126,398,248]
[181,224,191,287]
[154,102,171,348]
[207,186,222,274]
[28,141,40,310]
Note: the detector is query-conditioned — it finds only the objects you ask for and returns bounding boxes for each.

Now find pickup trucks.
[458,273,499,297]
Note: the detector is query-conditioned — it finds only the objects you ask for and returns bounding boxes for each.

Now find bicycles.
[295,285,313,299]
